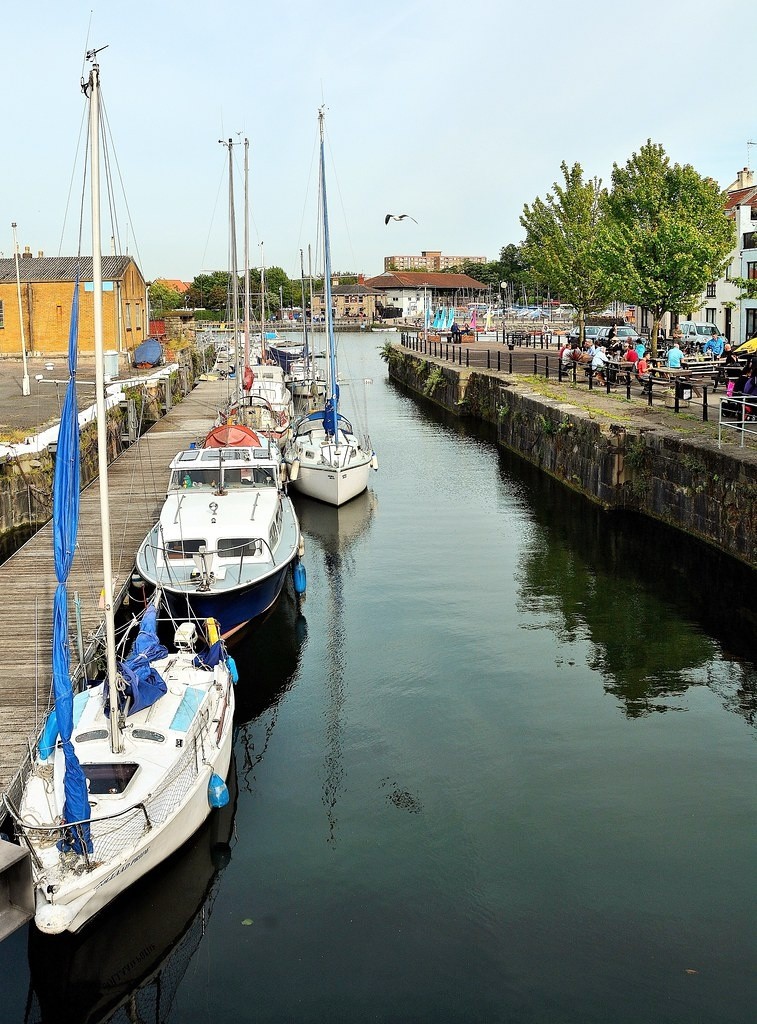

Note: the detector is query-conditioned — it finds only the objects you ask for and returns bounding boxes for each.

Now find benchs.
[561,339,757,413]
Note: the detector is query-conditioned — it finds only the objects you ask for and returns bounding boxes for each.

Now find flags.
[467,308,476,328]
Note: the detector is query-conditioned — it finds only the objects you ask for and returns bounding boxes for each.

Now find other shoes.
[600,382,605,386]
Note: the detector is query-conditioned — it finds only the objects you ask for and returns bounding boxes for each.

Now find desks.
[650,367,692,380]
[683,359,747,370]
[607,361,633,368]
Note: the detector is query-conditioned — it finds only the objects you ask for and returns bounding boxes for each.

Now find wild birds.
[385,213,419,226]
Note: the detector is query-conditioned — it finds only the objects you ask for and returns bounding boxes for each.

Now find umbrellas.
[733,337,757,356]
[419,303,455,330]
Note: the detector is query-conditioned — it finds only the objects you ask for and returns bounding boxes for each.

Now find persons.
[621,334,654,396]
[665,343,684,369]
[722,344,733,358]
[608,323,618,346]
[449,322,461,341]
[581,339,608,386]
[558,342,574,376]
[731,357,757,408]
[672,324,683,340]
[702,331,723,360]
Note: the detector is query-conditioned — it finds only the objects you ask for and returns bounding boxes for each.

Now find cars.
[567,325,650,349]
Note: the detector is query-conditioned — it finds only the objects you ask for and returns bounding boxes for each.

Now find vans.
[679,320,729,350]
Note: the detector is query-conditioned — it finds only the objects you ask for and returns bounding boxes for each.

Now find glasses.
[624,345,627,348]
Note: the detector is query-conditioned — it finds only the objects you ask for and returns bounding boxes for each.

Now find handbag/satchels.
[726,380,735,398]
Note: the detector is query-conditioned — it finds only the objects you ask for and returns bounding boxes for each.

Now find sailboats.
[294,487,376,826]
[20,742,238,1024]
[192,560,306,730]
[1,45,238,941]
[130,99,337,646]
[277,107,376,507]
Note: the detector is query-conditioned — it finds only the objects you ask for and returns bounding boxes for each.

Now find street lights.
[501,281,508,343]
